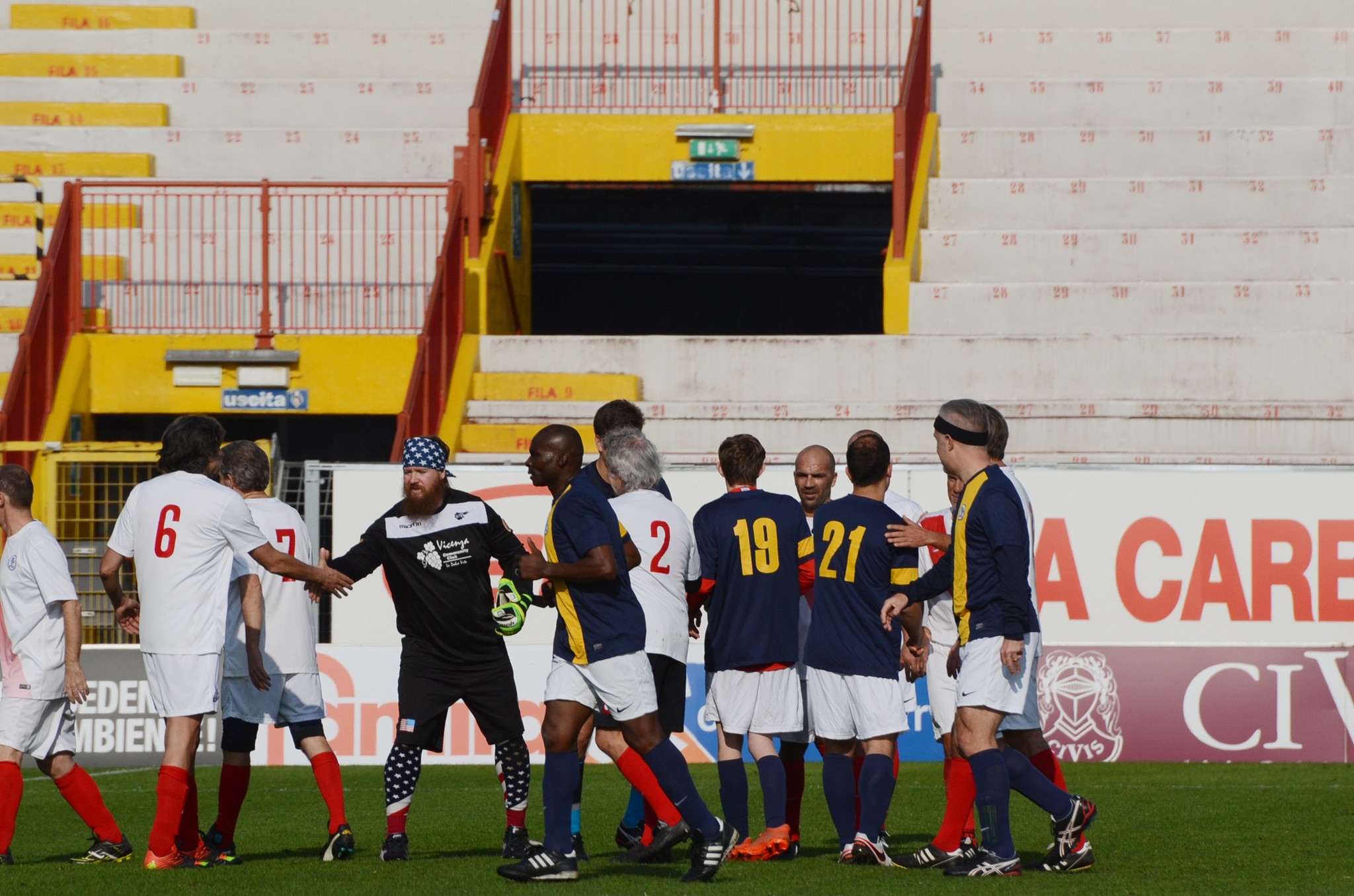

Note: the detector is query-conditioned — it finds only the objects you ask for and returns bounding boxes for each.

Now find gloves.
[491,578,533,636]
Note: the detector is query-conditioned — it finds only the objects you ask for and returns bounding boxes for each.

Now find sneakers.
[144,844,213,869]
[727,824,790,862]
[179,836,243,866]
[881,830,891,848]
[1026,840,1095,871]
[198,824,236,857]
[775,840,800,861]
[1043,795,1098,865]
[379,832,410,861]
[614,821,644,850]
[681,816,739,883]
[852,832,895,868]
[837,842,854,862]
[643,816,692,858]
[320,820,355,862]
[69,831,133,865]
[610,842,674,864]
[497,840,580,882]
[0,848,14,865]
[943,845,1022,876]
[502,825,529,859]
[960,837,980,861]
[891,843,964,869]
[571,832,589,860]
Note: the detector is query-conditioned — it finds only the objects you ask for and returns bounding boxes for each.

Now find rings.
[1013,658,1018,661]
[515,568,519,576]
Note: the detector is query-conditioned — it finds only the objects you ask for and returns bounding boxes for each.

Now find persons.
[98,418,355,870]
[0,463,131,867]
[495,399,1101,883]
[192,441,358,865]
[301,435,535,866]
